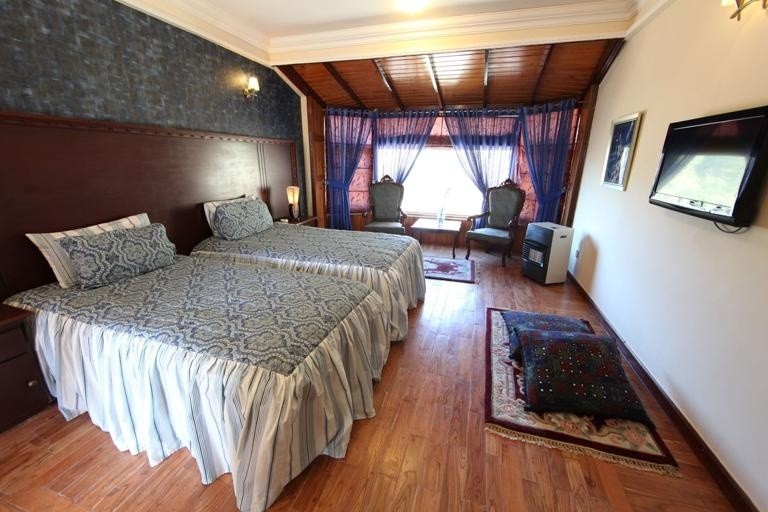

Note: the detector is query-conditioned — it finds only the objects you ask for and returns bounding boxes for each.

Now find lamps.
[242,76,260,101]
[285,185,299,219]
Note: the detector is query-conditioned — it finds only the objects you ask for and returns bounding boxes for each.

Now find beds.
[189,222,426,342]
[3,255,391,511]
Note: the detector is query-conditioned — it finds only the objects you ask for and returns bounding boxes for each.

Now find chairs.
[465,178,526,267]
[360,175,407,235]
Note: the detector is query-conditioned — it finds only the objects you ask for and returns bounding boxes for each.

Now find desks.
[410,217,462,259]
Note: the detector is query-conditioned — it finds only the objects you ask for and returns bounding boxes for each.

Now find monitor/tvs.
[648,106,768,232]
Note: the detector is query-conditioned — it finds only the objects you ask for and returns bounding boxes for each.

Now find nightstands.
[0,303,49,434]
[274,216,319,227]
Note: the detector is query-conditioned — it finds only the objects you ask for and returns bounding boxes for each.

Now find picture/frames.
[600,111,642,191]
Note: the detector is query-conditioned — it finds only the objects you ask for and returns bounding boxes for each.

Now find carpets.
[423,256,480,284]
[484,307,685,478]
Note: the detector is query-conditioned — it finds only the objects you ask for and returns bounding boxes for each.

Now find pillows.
[514,326,656,430]
[25,212,151,289]
[214,199,273,241]
[203,192,258,239]
[500,311,592,362]
[54,222,177,291]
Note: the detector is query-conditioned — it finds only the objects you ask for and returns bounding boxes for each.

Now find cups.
[280,219,288,223]
